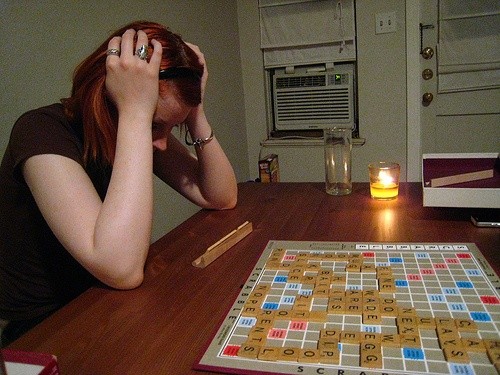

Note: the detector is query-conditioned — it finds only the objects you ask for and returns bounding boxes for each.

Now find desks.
[5,181,500,375]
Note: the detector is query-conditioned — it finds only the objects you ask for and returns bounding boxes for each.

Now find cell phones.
[471,216,500,227]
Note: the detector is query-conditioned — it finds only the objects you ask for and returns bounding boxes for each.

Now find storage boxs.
[421,153,500,208]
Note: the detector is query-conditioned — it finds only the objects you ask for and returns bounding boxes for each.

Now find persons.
[0,20,238,348]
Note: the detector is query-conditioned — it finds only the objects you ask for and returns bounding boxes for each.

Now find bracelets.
[185,126,213,149]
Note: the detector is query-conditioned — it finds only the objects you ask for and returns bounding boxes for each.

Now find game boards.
[192,239,500,375]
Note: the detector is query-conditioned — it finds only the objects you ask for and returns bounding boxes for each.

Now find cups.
[368,161,400,200]
[323,126,352,195]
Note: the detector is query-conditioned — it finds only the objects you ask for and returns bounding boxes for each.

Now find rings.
[135,44,148,60]
[106,48,121,56]
